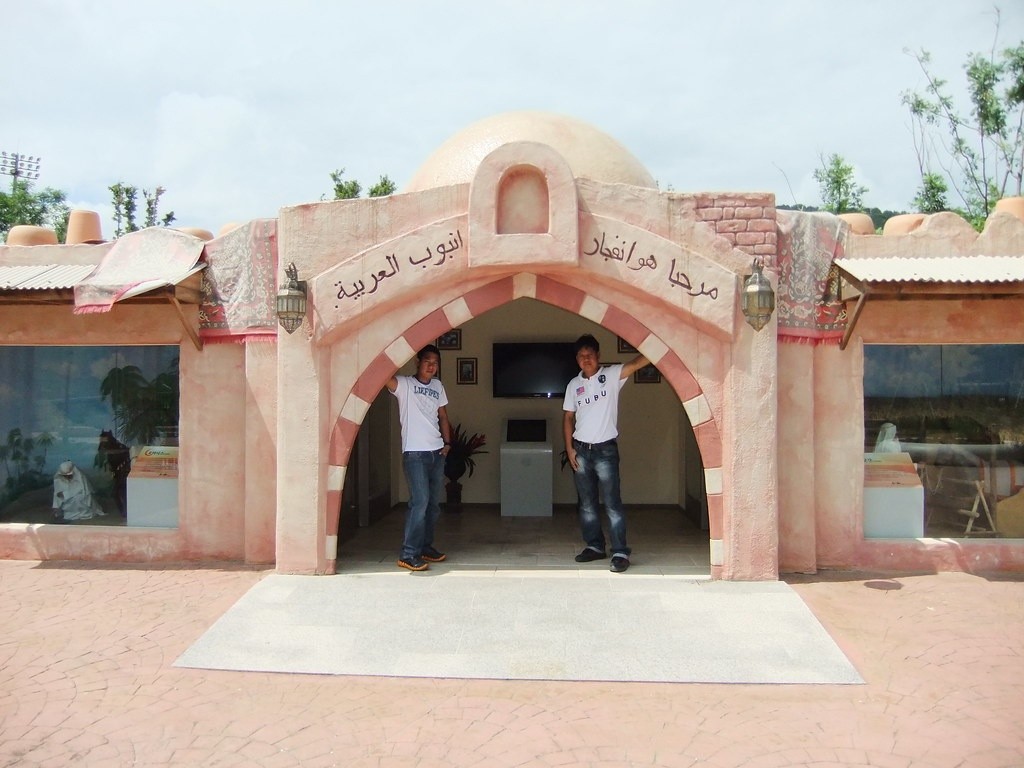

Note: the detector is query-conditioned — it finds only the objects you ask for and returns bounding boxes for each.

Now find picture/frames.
[457,357,477,385]
[618,336,639,353]
[598,362,622,368]
[430,357,441,383]
[634,362,661,383]
[436,329,461,350]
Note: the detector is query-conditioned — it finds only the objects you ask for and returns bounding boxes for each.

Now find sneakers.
[397,555,429,571]
[422,549,446,562]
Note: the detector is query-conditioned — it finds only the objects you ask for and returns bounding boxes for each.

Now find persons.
[873,423,927,474]
[53,462,104,520]
[562,334,651,572]
[384,344,452,572]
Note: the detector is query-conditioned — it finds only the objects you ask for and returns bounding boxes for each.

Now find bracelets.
[444,441,452,447]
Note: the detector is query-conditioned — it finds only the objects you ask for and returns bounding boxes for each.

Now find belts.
[571,437,617,451]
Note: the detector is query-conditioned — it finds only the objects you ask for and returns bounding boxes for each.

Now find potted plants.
[444,421,489,504]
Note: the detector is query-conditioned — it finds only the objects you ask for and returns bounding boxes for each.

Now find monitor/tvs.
[506,419,547,442]
[493,341,583,398]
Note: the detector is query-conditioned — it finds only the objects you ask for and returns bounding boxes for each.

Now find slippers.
[574,546,606,563]
[609,553,630,572]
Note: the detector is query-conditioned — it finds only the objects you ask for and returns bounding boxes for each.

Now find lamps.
[741,258,775,331]
[277,261,306,336]
